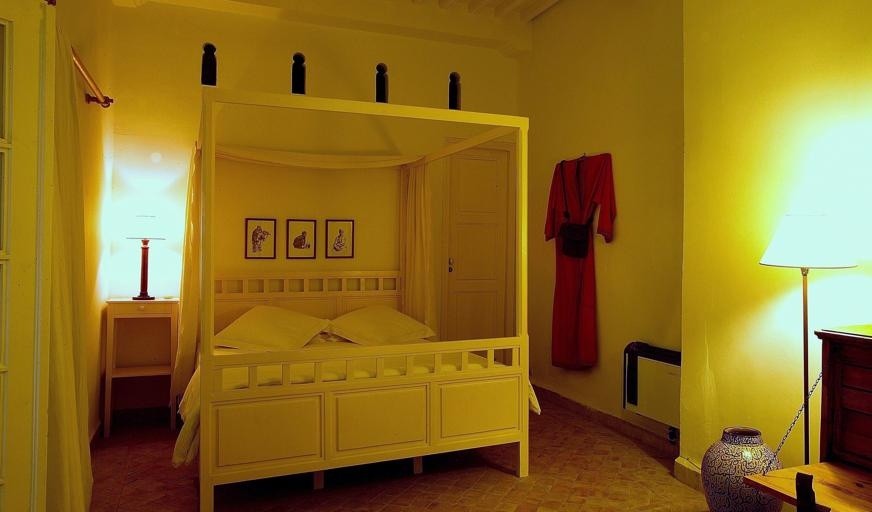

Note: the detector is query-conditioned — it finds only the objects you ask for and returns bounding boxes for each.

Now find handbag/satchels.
[560,223,589,257]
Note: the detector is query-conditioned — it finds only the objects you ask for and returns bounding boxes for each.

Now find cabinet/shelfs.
[744,322,872,512]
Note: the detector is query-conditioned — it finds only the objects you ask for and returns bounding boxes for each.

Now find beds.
[173,43,544,512]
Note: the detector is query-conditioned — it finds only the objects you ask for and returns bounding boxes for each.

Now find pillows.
[214,304,328,351]
[323,304,436,346]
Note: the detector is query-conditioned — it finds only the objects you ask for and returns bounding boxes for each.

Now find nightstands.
[103,299,177,437]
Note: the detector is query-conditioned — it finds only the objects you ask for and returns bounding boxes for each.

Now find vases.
[700,426,783,512]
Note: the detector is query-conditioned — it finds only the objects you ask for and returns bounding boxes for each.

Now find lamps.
[759,213,860,464]
[126,214,166,300]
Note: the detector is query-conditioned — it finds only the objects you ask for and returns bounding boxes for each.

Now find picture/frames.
[244,218,276,260]
[286,218,317,259]
[324,218,354,259]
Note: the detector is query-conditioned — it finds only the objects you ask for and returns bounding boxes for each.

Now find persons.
[293,231,311,249]
[252,225,268,252]
[332,228,347,251]
